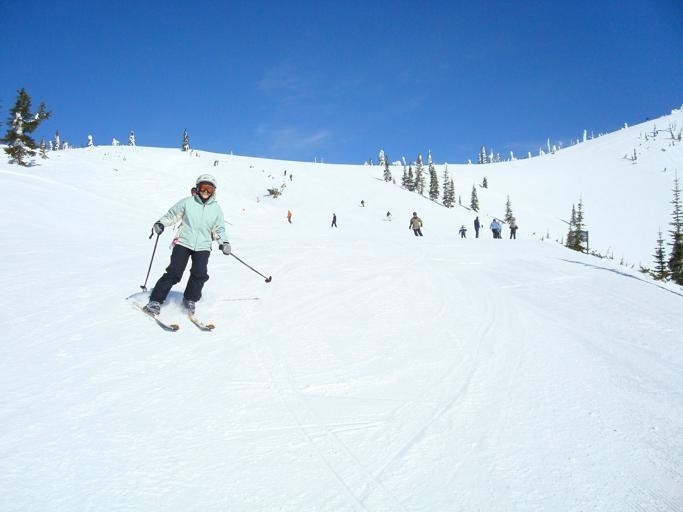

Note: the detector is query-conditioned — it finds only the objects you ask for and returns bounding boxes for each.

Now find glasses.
[196,184,215,194]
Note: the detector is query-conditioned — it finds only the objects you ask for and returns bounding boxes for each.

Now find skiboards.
[135,298,214,332]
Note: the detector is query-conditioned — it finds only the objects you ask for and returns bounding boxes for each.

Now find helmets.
[195,174,216,188]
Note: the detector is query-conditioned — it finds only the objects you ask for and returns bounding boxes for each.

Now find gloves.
[218,242,231,255]
[153,220,164,235]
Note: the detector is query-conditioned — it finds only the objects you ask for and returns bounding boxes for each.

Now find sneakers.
[182,296,195,310]
[145,299,161,315]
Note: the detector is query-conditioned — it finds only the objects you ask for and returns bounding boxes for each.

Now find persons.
[331,213,337,228]
[459,216,518,239]
[144,173,231,315]
[387,211,392,216]
[361,199,365,207]
[287,209,292,223]
[409,211,424,236]
